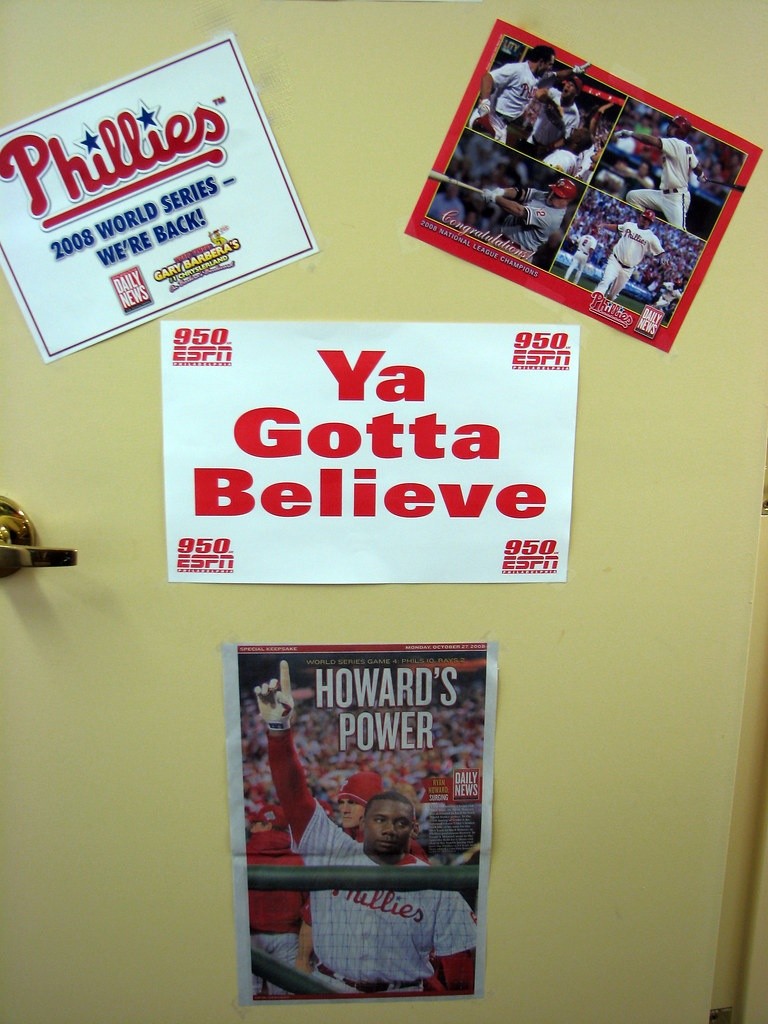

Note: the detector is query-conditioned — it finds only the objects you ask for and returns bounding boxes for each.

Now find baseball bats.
[708,178,746,193]
[428,170,484,195]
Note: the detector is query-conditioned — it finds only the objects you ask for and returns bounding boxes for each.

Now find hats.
[337,772,383,808]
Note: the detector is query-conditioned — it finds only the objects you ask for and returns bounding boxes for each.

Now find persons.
[426,45,747,329]
[238,659,477,993]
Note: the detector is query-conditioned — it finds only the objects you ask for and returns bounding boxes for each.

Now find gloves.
[481,187,505,204]
[478,99,491,116]
[613,130,634,137]
[573,64,585,74]
[253,660,294,730]
[697,172,708,182]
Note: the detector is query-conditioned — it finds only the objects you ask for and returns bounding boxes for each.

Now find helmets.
[591,227,598,235]
[641,209,655,223]
[549,179,577,199]
[570,76,583,94]
[669,115,689,140]
[675,279,681,286]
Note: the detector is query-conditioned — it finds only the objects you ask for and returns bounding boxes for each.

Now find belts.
[614,254,631,268]
[662,297,667,300]
[577,249,588,255]
[317,964,422,993]
[663,188,678,194]
[498,233,533,253]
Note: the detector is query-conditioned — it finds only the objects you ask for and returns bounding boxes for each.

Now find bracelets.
[627,131,634,136]
[481,99,490,106]
